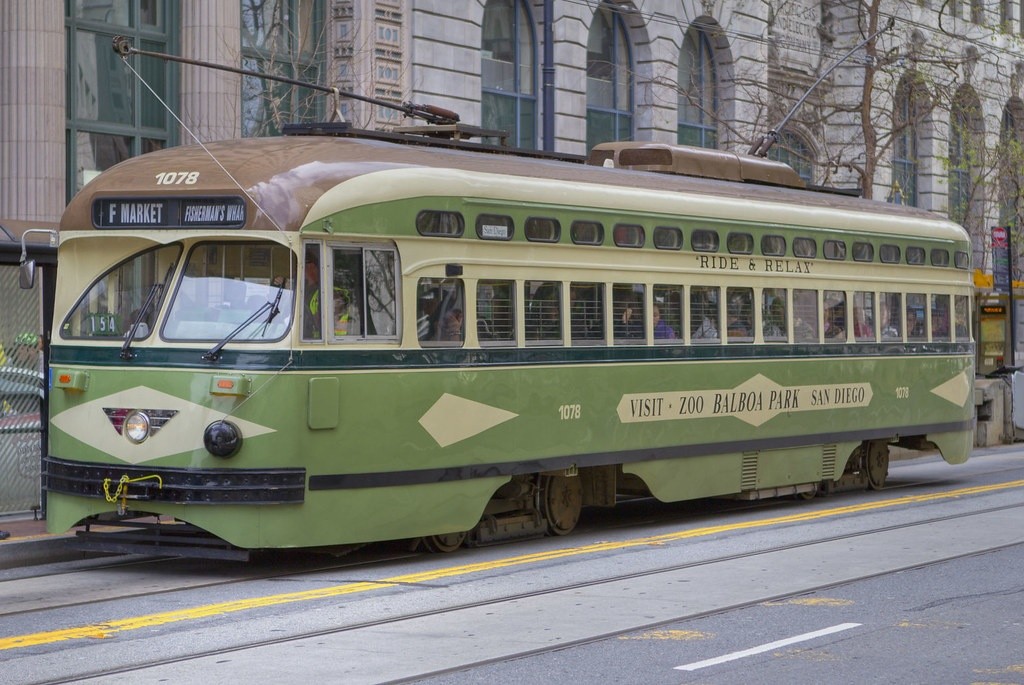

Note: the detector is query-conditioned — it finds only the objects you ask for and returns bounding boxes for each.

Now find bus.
[19,116,978,571]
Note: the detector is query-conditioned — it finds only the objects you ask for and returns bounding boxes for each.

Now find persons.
[115,290,269,340]
[269,244,352,338]
[1,333,44,417]
[418,287,461,342]
[473,280,971,338]
